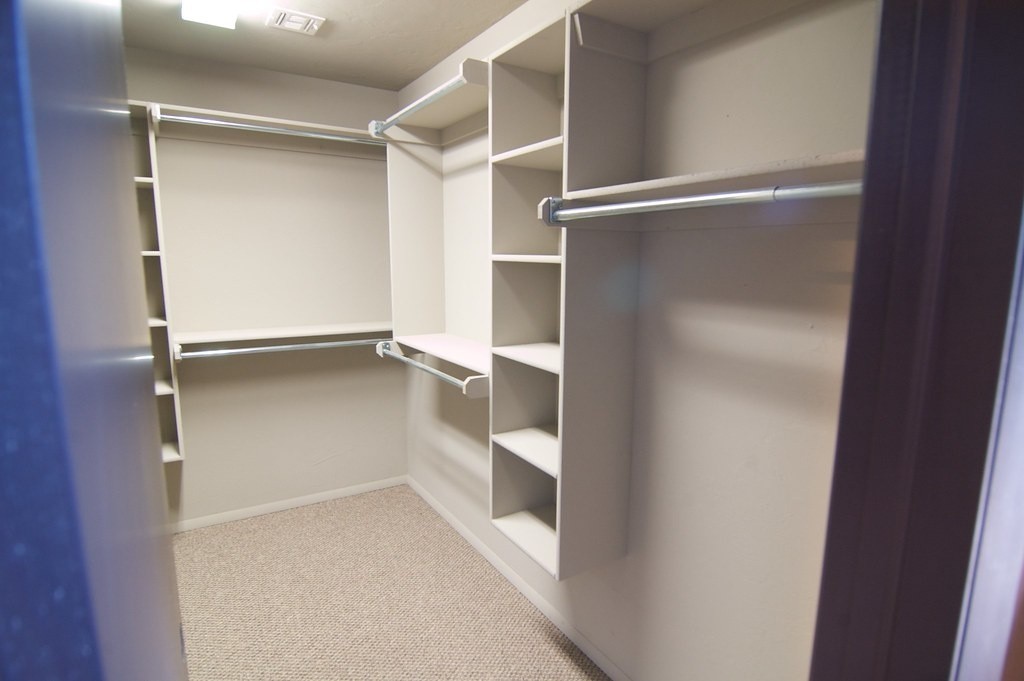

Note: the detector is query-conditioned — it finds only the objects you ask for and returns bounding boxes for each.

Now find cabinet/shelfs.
[124,1,875,584]
